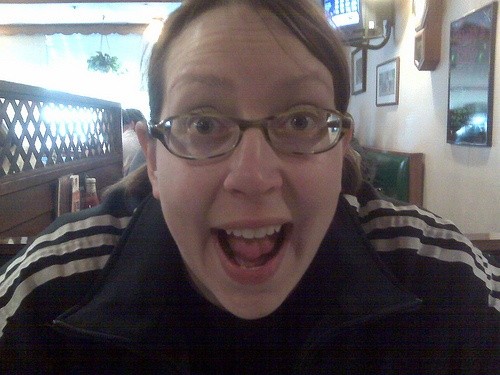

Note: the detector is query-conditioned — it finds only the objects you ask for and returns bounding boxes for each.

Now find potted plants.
[87,53,120,74]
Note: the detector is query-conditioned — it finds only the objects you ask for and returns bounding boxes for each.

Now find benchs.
[363,145,425,207]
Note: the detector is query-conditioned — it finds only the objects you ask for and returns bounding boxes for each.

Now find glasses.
[149,104,352,160]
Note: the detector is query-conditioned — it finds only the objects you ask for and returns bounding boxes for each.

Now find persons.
[101,108,150,177]
[0,0,499,375]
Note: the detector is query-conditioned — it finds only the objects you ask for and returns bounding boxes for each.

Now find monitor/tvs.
[323,0,377,33]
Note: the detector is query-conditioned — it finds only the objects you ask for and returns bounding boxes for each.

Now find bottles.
[83,178,100,208]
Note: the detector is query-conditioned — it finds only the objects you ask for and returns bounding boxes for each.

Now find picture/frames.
[350,47,367,96]
[446,1,498,147]
[376,57,400,107]
